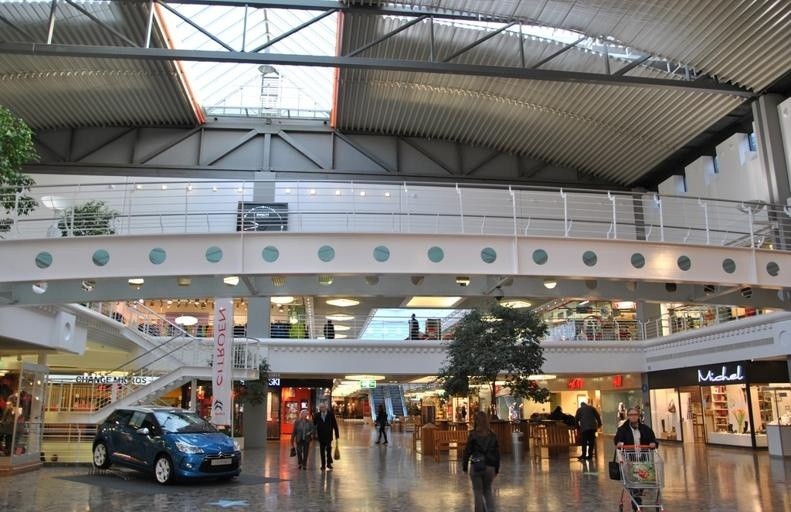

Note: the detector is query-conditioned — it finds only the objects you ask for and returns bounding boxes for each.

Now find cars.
[91,406,241,486]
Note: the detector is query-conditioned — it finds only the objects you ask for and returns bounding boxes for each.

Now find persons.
[324,320,335,339]
[549,406,566,420]
[462,412,500,512]
[290,410,315,470]
[375,404,388,444]
[613,408,659,512]
[616,402,627,427]
[313,404,339,470]
[574,402,602,460]
[408,314,419,340]
[0,391,41,458]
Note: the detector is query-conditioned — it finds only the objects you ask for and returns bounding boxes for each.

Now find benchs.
[412,417,598,462]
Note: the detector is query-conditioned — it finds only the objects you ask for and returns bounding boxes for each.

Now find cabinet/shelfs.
[711,383,774,433]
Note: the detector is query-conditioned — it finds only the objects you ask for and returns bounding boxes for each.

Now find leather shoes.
[578,456,591,460]
[321,465,333,470]
[298,466,306,469]
[376,442,388,444]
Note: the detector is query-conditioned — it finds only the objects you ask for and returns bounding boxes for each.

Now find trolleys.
[615,444,666,511]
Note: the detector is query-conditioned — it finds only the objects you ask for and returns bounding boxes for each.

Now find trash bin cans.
[271,323,290,338]
[719,306,732,323]
[427,319,443,340]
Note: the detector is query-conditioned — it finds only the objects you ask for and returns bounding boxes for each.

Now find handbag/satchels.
[609,461,620,480]
[290,448,295,457]
[335,447,340,459]
[471,456,485,471]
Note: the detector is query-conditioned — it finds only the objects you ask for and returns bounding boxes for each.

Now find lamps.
[543,277,558,291]
[500,298,530,310]
[107,297,299,329]
[455,275,472,287]
[128,278,145,290]
[223,276,240,286]
[400,295,468,309]
[402,374,557,399]
[612,300,637,311]
[316,297,361,340]
[330,375,386,398]
[33,281,47,294]
